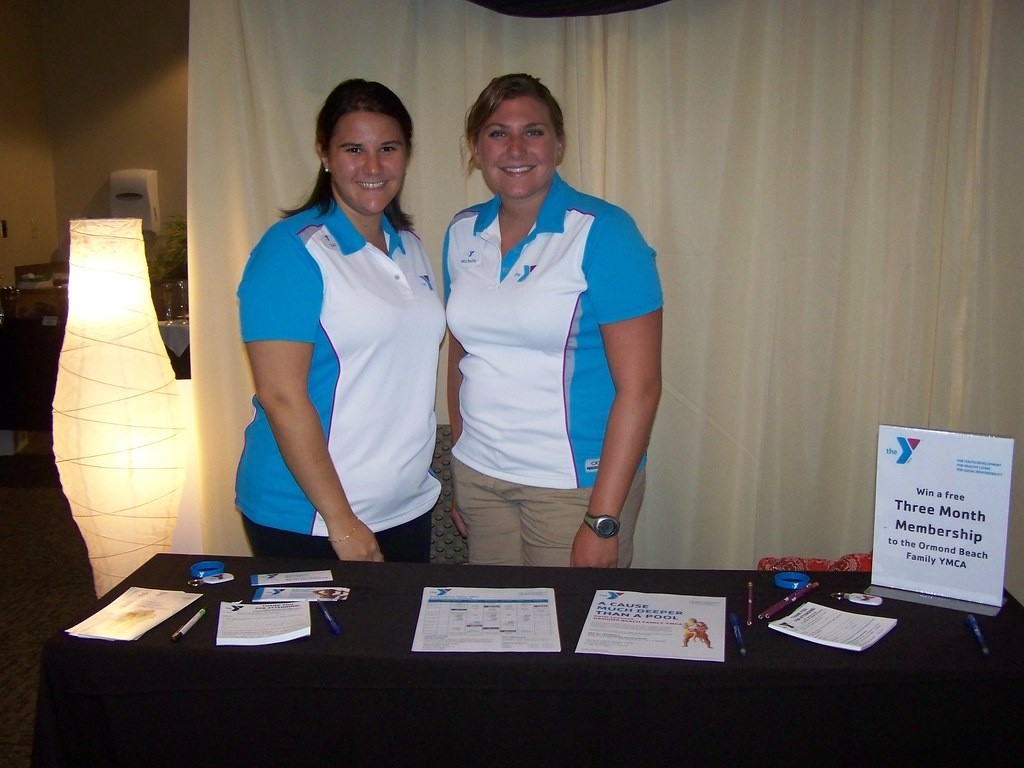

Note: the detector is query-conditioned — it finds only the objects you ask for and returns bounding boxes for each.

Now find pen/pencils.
[764,582,819,619]
[171,606,208,642]
[318,599,342,636]
[730,612,747,657]
[747,581,753,626]
[967,614,989,657]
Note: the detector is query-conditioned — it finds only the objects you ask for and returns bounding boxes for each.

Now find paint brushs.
[758,583,812,619]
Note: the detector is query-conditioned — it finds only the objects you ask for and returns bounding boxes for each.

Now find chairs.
[430,424,469,564]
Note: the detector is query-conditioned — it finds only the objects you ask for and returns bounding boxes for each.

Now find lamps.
[50,218,190,603]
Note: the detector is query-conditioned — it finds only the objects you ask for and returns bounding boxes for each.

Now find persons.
[443,73,665,568]
[235,78,442,565]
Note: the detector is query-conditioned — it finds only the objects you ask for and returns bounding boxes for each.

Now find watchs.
[582,511,621,539]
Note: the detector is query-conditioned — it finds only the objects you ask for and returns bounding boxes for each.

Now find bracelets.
[327,518,360,542]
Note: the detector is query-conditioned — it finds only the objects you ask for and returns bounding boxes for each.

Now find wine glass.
[162,283,174,326]
[175,280,189,325]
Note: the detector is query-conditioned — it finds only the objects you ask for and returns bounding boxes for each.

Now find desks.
[34,549,1024,768]
[158,318,190,379]
